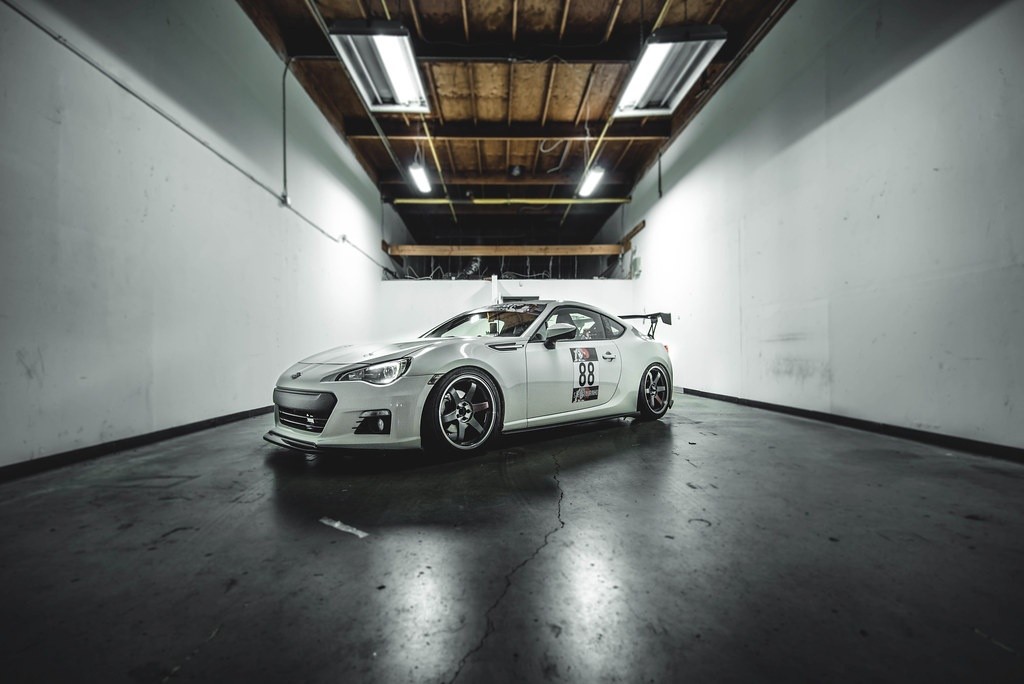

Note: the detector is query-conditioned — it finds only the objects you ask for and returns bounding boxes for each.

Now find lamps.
[610,1,727,118]
[408,120,431,192]
[576,100,605,197]
[328,0,432,114]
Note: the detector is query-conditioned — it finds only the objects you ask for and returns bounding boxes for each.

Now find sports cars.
[263,297,674,462]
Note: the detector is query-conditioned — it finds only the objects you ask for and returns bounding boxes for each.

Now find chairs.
[555,314,580,339]
